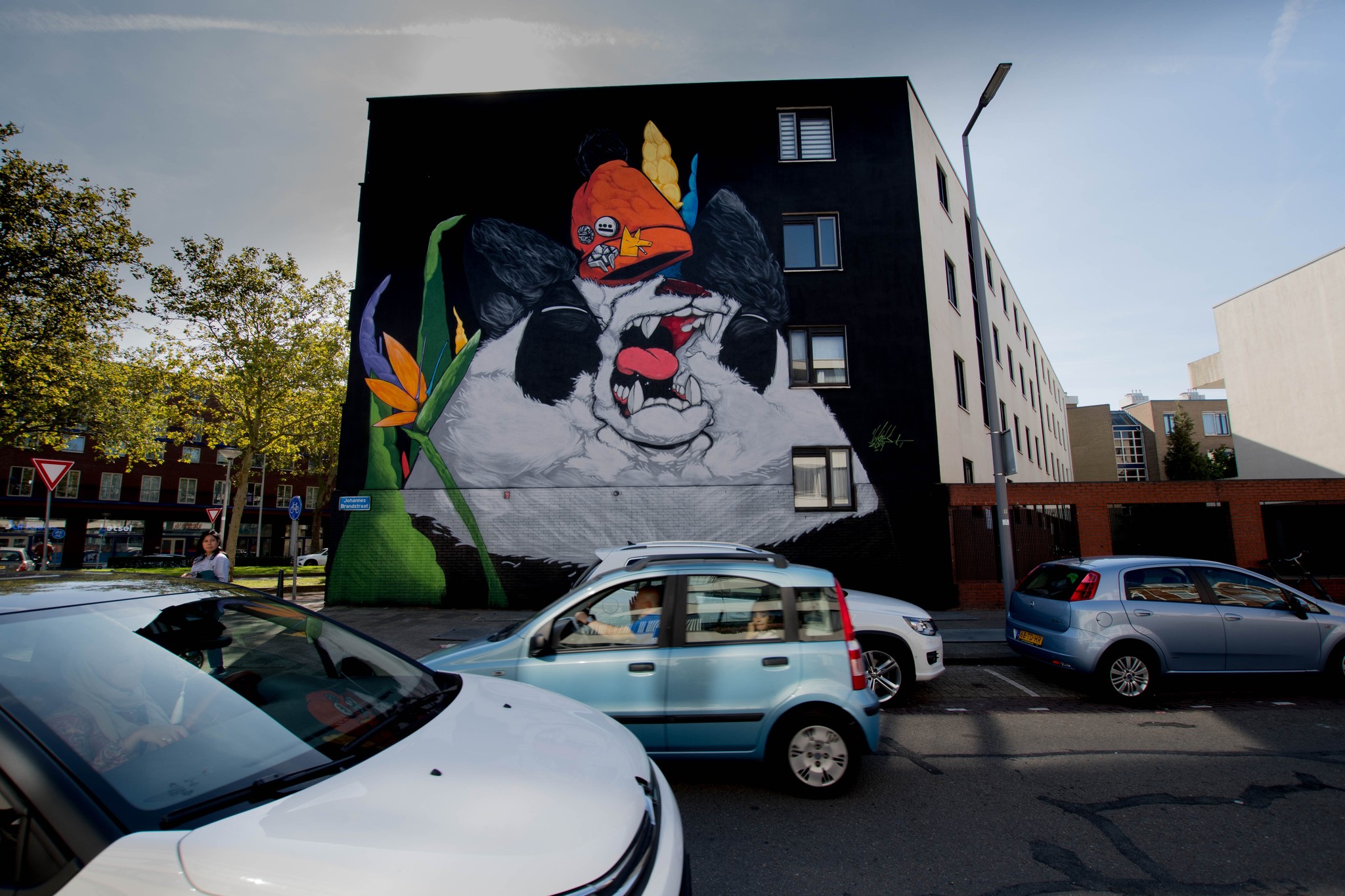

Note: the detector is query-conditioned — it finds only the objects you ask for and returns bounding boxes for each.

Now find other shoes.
[208,666,227,676]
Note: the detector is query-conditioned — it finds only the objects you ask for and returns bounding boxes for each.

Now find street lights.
[219,447,243,550]
[961,63,1016,615]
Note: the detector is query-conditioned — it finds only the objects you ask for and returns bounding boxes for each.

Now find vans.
[136,554,187,568]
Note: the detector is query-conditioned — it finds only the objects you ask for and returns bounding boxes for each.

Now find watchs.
[585,615,593,625]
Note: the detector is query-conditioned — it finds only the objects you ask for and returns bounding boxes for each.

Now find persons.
[747,600,782,639]
[34,538,54,571]
[575,585,662,645]
[181,529,230,675]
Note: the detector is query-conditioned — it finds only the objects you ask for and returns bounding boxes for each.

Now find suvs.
[571,539,946,714]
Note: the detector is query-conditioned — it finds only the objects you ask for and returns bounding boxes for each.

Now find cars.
[0,568,693,896]
[0,547,36,572]
[290,548,329,567]
[416,554,880,801]
[1006,555,1345,710]
